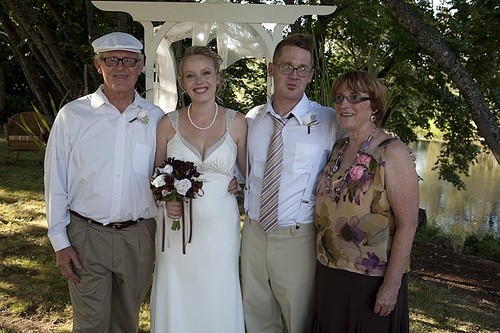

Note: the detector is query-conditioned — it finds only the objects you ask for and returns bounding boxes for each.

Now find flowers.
[150,157,203,232]
[301,111,317,135]
[128,108,150,125]
[349,153,379,181]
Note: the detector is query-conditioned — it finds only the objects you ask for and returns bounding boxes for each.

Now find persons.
[308,71,420,333]
[150,46,248,333]
[43,32,241,333]
[238,34,343,333]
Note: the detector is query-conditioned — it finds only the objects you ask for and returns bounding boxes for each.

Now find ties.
[260,112,295,235]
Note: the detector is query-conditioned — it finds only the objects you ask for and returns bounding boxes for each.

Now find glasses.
[273,61,313,78]
[102,56,142,67]
[331,92,371,104]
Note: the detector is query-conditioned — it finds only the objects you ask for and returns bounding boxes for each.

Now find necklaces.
[188,102,218,130]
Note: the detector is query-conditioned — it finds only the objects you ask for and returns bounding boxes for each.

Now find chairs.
[4,112,48,165]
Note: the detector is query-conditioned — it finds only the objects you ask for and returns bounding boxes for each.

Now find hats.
[92,32,143,54]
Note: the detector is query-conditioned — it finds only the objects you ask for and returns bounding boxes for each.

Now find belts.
[69,209,144,230]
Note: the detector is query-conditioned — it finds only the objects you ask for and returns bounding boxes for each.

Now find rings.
[388,309,391,312]
